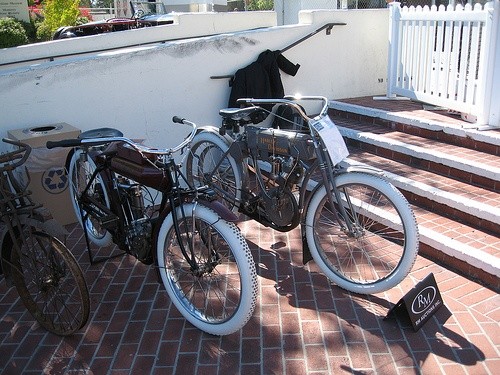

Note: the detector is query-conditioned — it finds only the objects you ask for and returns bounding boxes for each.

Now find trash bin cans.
[7,122,86,228]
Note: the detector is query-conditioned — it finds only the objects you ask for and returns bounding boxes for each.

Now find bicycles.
[0,138,90,336]
[70,113,259,337]
[187,96,420,291]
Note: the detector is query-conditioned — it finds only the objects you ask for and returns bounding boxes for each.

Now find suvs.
[53,0,181,40]
